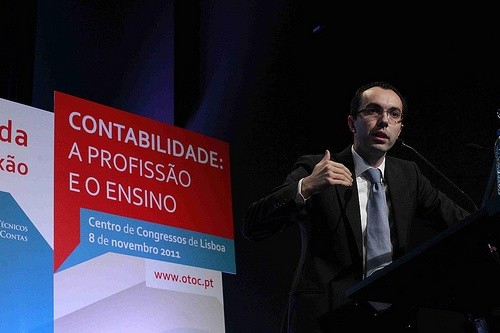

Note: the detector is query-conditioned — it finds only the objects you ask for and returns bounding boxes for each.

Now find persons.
[241,80,496,332]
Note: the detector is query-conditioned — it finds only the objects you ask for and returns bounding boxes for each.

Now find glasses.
[361,108,403,121]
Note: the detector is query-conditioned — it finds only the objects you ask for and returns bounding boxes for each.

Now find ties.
[366,168,392,311]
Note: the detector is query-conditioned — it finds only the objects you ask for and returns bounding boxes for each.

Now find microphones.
[398,139,479,212]
